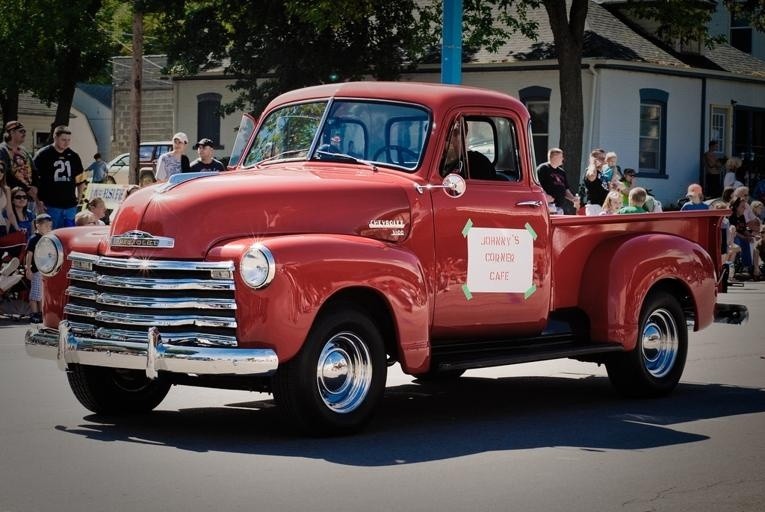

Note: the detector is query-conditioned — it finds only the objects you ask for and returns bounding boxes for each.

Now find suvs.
[138,140,174,188]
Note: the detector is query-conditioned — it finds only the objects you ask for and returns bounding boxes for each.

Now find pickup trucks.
[24,80,733,440]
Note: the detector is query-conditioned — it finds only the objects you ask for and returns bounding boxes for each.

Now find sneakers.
[29,312,43,323]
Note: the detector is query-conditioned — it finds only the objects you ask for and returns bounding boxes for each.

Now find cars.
[104,153,130,186]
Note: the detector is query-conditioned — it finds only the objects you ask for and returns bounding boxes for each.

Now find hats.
[194,138,214,148]
[685,184,702,197]
[172,132,188,144]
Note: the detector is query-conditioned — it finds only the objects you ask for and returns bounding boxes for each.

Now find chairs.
[703,153,725,193]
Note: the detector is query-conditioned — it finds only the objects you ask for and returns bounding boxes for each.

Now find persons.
[537,139,764,286]
[262,141,281,162]
[315,136,343,162]
[346,140,364,160]
[0,120,109,325]
[444,119,497,181]
[190,138,225,171]
[155,132,191,181]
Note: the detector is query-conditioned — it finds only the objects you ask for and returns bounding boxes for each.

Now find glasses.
[0,167,6,172]
[15,195,28,199]
